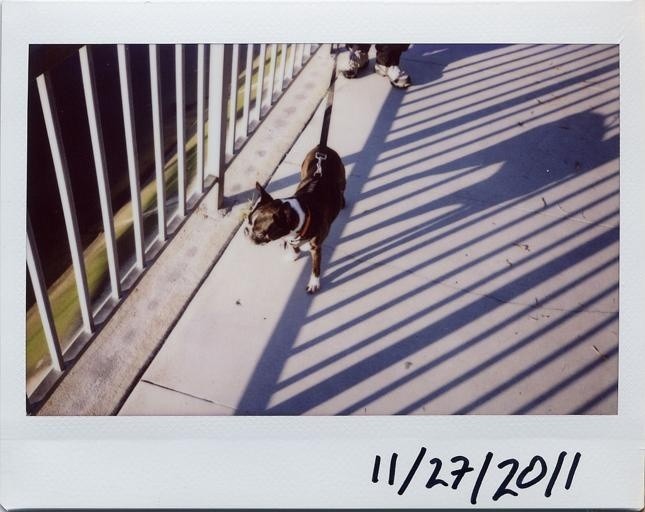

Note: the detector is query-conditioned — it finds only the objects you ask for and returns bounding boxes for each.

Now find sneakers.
[374,63,411,89]
[342,50,369,78]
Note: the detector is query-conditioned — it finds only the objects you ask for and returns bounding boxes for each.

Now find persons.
[341,44,412,89]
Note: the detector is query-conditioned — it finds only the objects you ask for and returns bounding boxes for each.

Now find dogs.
[244,144,346,294]
